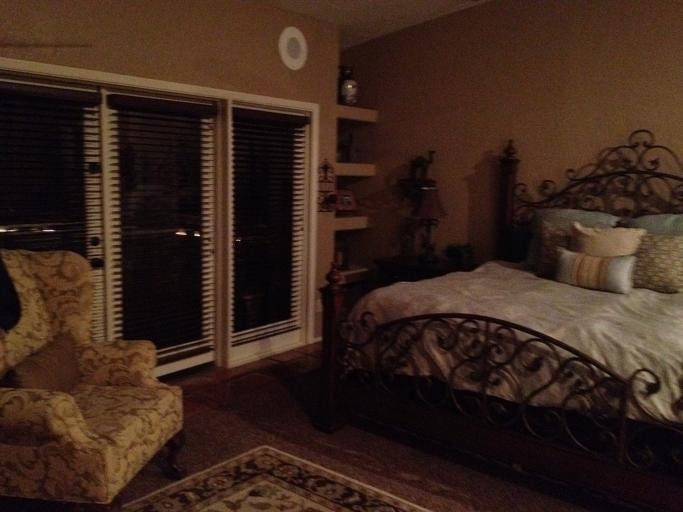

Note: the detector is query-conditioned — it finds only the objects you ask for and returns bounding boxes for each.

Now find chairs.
[0,246,188,509]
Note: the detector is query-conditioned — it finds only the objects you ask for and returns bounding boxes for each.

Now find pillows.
[1,328,83,394]
[525,206,682,296]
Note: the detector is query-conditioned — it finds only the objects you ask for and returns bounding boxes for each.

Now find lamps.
[410,180,449,262]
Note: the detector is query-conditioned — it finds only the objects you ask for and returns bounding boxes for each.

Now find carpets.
[118,443,433,511]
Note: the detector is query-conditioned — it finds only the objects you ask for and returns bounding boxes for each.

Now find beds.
[312,126,682,510]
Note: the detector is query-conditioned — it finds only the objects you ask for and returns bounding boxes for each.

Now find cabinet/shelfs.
[335,102,381,287]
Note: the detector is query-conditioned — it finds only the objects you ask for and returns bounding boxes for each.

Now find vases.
[338,64,362,106]
